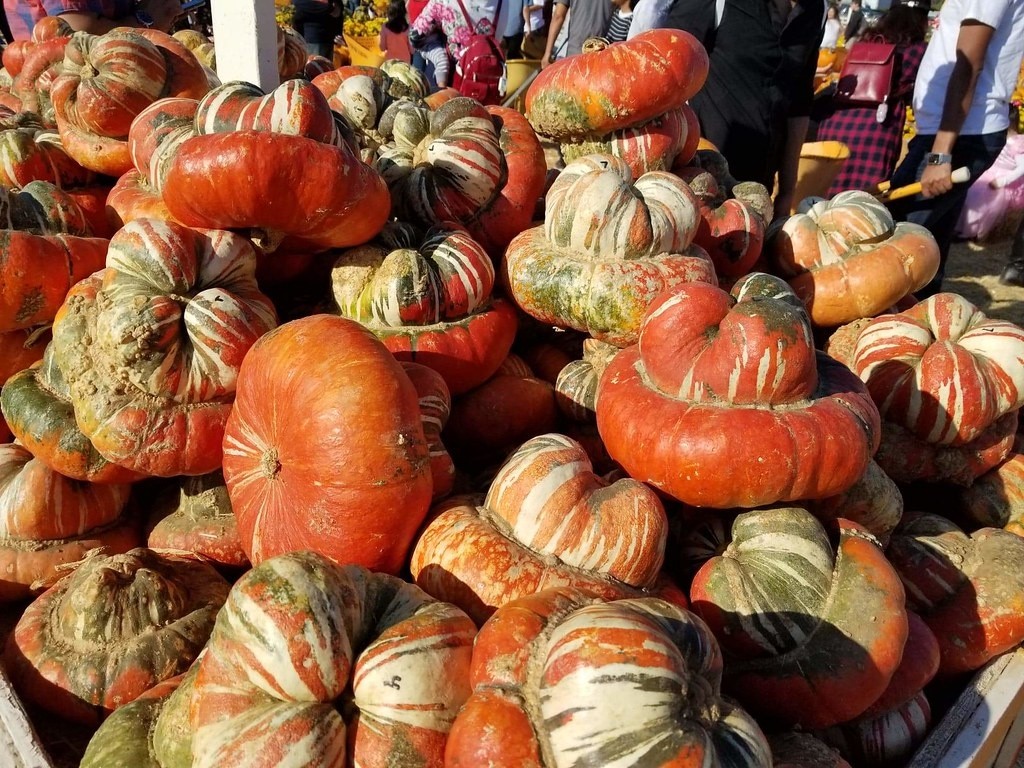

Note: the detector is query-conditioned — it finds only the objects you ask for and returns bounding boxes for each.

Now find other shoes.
[999,257,1024,285]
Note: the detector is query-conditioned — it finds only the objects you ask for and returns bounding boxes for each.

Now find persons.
[1,0,1024,302]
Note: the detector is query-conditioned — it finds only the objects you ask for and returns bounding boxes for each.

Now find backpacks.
[452,0,506,106]
[835,34,899,123]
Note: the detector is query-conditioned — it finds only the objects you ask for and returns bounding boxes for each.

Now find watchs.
[926,152,952,166]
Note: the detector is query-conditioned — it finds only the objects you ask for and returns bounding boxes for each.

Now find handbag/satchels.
[520,33,547,59]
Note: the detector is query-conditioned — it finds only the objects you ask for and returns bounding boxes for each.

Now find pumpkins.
[0,15,1024,768]
